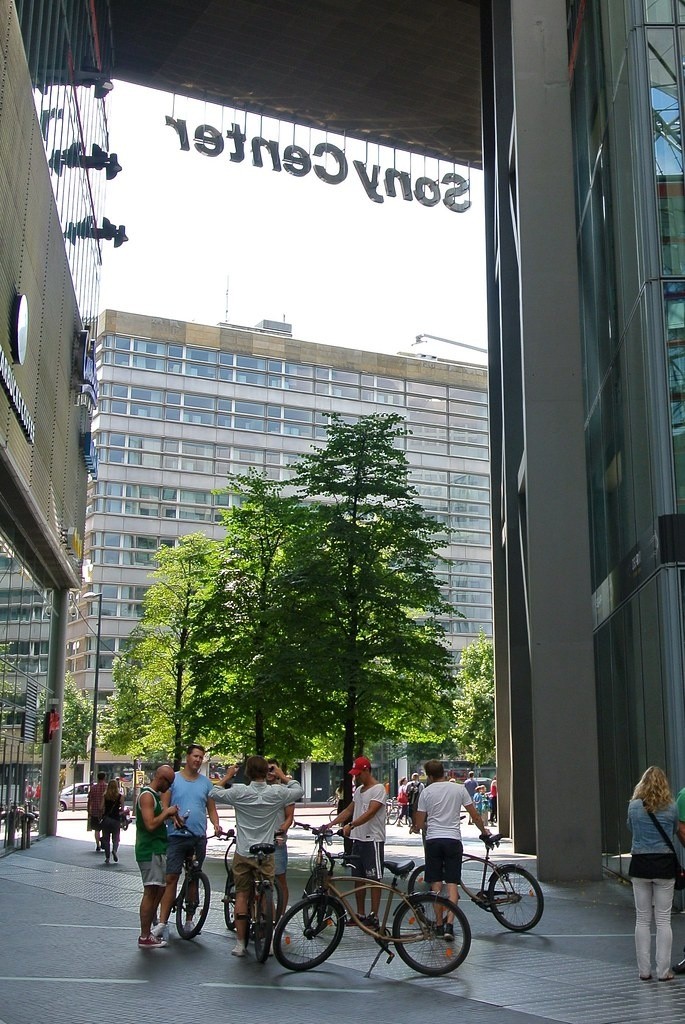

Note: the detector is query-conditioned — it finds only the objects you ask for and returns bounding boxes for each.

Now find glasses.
[190,743,206,755]
[164,776,172,786]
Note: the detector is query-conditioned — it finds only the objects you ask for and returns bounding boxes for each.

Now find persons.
[444,771,497,826]
[627,766,685,981]
[135,745,222,948]
[324,757,386,933]
[383,773,425,834]
[209,756,305,956]
[412,760,491,940]
[85,788,88,794]
[25,779,42,811]
[335,779,344,815]
[87,770,124,862]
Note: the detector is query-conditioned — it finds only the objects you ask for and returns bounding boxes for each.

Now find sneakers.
[183,921,202,934]
[151,923,166,937]
[138,934,166,947]
[261,938,275,956]
[231,944,247,956]
[150,920,163,937]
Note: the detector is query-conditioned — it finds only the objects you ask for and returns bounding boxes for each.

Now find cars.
[57,782,98,812]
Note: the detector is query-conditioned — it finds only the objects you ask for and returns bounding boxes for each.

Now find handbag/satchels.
[87,810,92,831]
[675,866,685,890]
[397,786,409,803]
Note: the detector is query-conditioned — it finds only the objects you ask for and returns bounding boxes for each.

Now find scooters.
[118,807,133,830]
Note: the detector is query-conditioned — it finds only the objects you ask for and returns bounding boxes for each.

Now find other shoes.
[111,849,118,862]
[468,823,474,825]
[345,913,364,926]
[397,824,403,826]
[104,858,110,863]
[658,973,674,981]
[640,974,652,980]
[444,922,455,942]
[435,924,444,938]
[491,824,497,827]
[365,912,379,929]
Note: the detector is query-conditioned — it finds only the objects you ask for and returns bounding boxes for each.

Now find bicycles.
[329,808,338,821]
[385,797,400,825]
[294,821,344,932]
[152,821,235,940]
[408,814,544,932]
[273,845,472,978]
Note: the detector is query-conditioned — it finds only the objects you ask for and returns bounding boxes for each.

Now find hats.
[349,756,371,775]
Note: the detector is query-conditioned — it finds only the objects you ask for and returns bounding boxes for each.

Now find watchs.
[349,822,355,830]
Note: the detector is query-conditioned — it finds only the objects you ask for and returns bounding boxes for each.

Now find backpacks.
[408,780,421,805]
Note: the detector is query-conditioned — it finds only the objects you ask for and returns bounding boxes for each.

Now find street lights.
[82,590,103,788]
